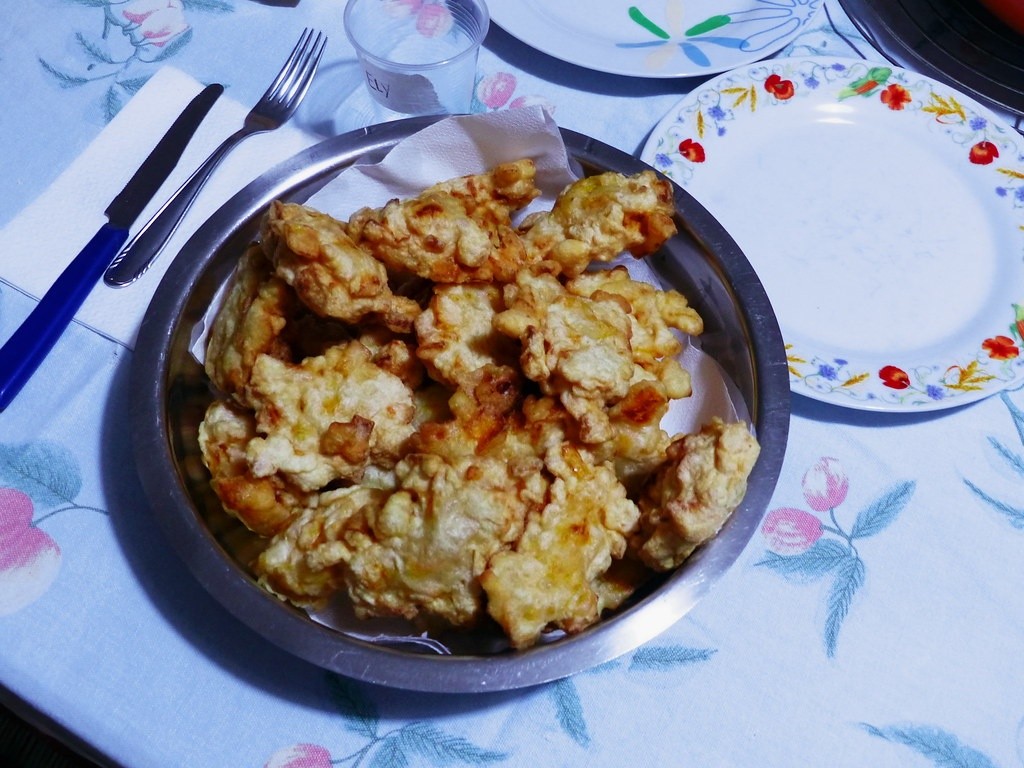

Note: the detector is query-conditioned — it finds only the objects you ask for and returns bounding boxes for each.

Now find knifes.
[0,84,224,414]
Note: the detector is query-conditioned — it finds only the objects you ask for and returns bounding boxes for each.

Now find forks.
[104,28,328,289]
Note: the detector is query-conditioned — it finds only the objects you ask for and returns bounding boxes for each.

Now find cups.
[342,0,490,121]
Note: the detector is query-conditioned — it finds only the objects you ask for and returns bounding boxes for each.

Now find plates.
[486,1,825,79]
[640,57,1024,414]
[127,112,792,695]
